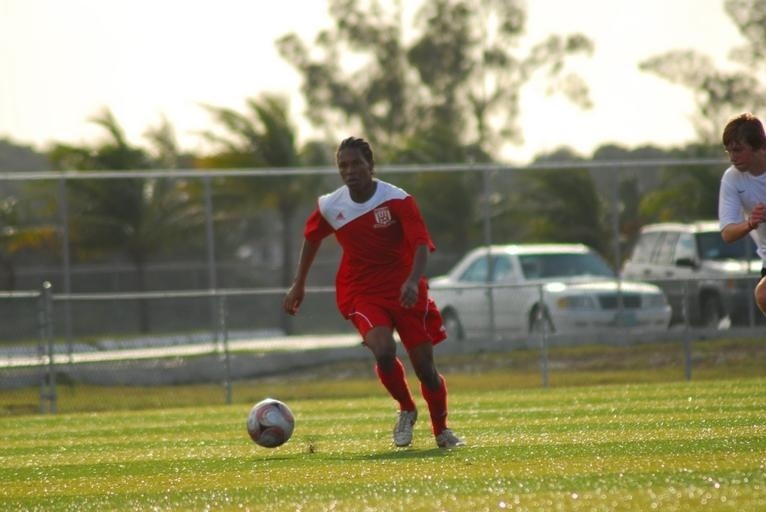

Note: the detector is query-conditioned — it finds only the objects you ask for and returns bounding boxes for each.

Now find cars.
[421,241,674,340]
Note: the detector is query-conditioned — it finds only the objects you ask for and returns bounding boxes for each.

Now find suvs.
[617,218,766,330]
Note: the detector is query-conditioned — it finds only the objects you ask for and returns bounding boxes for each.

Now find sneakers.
[436,429,464,448]
[393,409,417,447]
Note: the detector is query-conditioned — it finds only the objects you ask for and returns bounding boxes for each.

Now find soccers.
[248,399,293,447]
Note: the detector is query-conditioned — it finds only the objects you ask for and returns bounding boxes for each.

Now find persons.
[282,137,470,449]
[717,113,766,319]
